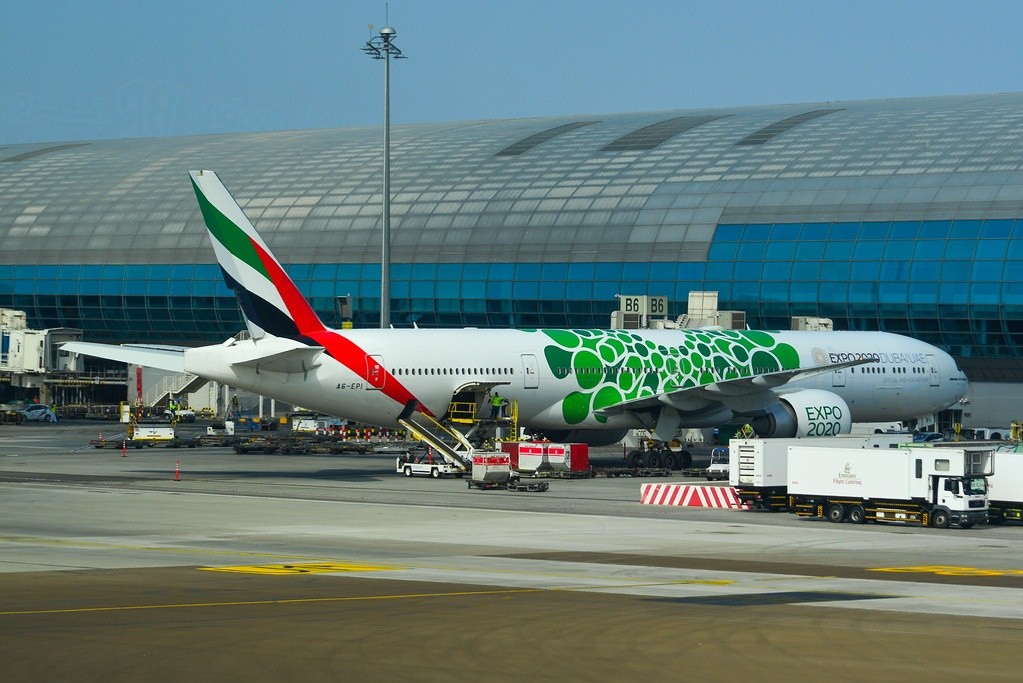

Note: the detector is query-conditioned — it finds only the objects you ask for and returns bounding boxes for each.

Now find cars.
[706,461,729,481]
[14,404,52,422]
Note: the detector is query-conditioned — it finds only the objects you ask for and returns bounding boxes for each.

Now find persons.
[33,396,39,404]
[170,401,177,415]
[713,427,720,445]
[737,423,751,439]
[488,391,506,419]
[49,402,58,423]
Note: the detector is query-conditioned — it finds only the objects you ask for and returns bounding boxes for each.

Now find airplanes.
[58,171,969,471]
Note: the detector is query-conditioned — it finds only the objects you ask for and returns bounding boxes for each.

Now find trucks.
[729,429,1023,527]
